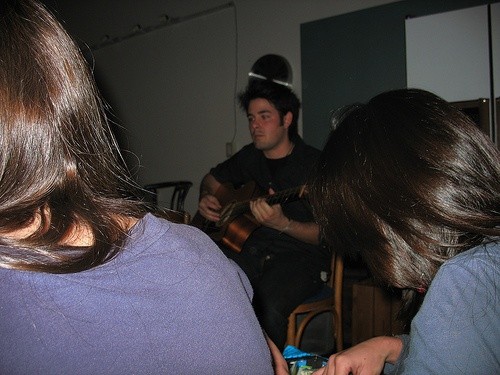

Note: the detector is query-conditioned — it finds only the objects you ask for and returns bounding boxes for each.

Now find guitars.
[191,171,309,255]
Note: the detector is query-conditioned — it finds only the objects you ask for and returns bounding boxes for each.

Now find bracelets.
[281,219,291,232]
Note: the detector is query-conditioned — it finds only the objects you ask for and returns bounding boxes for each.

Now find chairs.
[140,181,193,211]
[287,251,344,352]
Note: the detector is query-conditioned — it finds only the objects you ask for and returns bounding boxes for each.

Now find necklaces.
[266,143,294,158]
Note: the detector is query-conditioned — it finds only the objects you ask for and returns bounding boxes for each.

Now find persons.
[303,88,500,375]
[196,80,329,355]
[0,0,277,375]
